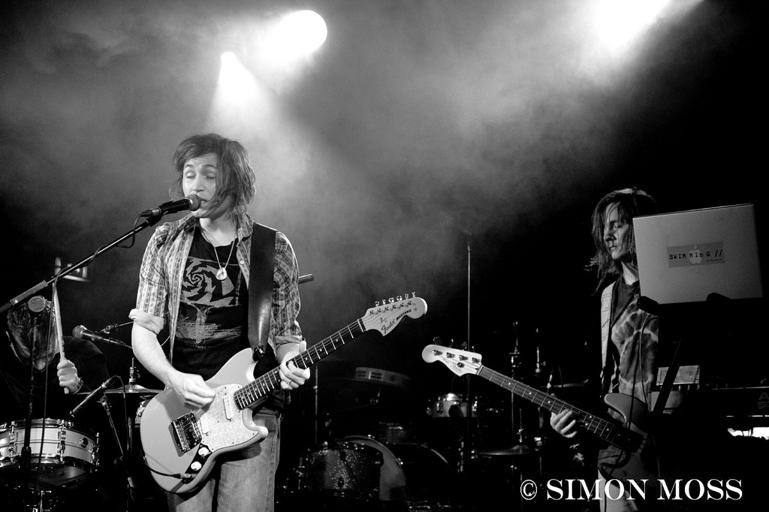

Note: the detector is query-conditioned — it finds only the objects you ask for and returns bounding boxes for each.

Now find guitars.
[140,290,428,496]
[421,336,694,510]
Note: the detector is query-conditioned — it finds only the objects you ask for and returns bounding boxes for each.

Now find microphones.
[69,378,116,418]
[140,194,201,217]
[72,324,124,347]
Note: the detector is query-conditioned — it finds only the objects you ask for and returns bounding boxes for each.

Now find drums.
[295,435,407,504]
[0,417,101,494]
[354,366,407,386]
[430,392,478,419]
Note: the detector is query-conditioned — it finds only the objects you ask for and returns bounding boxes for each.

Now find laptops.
[631,203,763,304]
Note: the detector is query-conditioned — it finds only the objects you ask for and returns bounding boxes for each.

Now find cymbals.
[78,385,162,395]
[317,357,347,364]
[480,452,519,456]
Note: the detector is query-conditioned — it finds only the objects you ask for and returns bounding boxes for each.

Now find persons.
[549,189,711,512]
[129,133,311,512]
[0,297,110,459]
